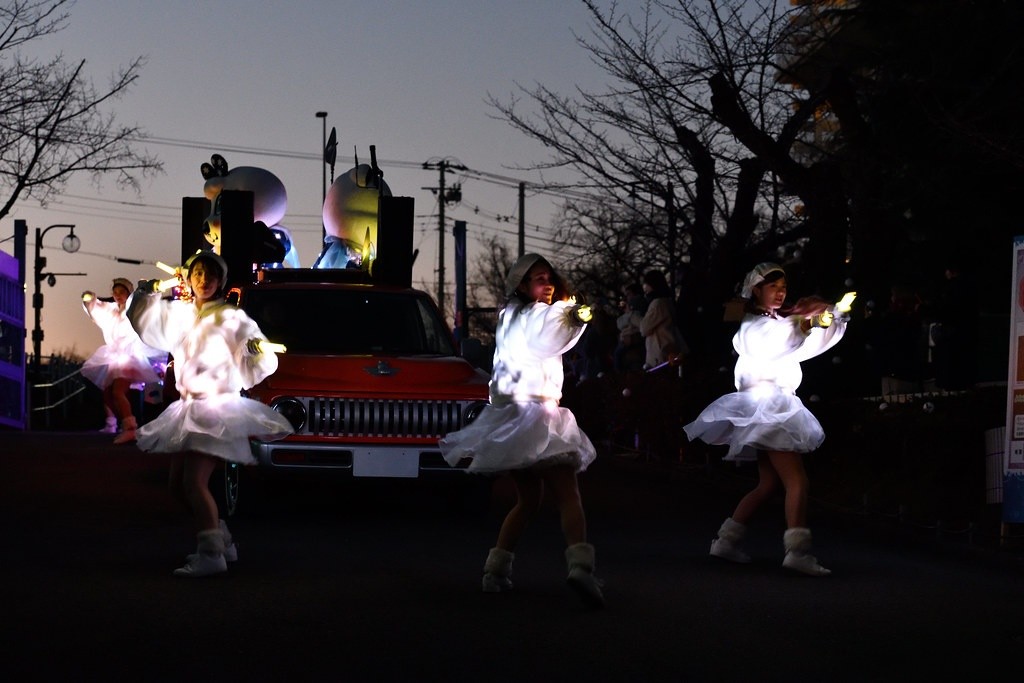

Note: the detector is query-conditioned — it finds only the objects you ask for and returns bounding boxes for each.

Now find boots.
[781,528,834,579]
[564,542,606,604]
[712,521,753,562]
[172,522,236,578]
[114,417,138,445]
[99,417,117,434]
[484,548,517,592]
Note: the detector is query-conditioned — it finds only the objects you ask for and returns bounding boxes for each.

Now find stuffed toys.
[198,152,291,270]
[312,164,394,271]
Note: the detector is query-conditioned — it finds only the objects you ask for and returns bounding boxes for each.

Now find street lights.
[33,222,79,381]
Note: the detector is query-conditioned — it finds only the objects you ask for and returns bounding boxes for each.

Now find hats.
[113,277,134,294]
[506,253,541,296]
[180,251,229,293]
[741,261,785,299]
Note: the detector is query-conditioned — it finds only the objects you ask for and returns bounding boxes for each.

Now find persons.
[79,276,164,445]
[438,252,860,609]
[125,253,282,539]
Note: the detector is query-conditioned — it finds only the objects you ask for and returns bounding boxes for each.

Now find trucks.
[213,268,495,514]
